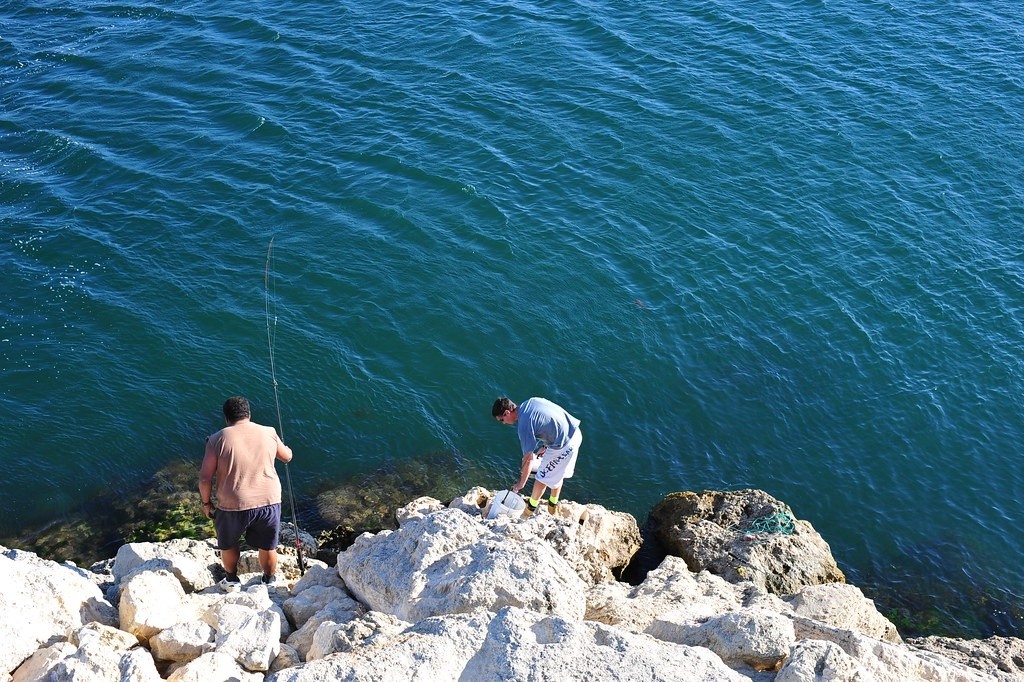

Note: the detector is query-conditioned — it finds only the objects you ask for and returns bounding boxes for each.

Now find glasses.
[499,412,506,423]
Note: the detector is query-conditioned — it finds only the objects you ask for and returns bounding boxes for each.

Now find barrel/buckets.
[487,489,526,519]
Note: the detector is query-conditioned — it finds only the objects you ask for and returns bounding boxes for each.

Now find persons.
[491,396,583,518]
[198,395,293,593]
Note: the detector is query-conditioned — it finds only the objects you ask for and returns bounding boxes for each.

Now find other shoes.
[260,574,278,589]
[219,577,241,592]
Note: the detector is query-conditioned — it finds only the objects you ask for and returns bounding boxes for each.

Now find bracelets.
[201,499,213,507]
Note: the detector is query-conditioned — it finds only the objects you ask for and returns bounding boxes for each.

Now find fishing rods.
[264,231,307,576]
